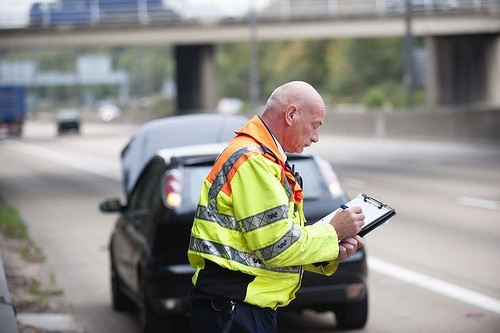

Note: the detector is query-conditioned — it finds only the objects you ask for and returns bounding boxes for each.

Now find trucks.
[0,86,27,139]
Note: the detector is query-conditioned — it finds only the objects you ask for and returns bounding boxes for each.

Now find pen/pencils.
[340,204,349,210]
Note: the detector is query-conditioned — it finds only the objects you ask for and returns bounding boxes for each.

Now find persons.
[186,80,367,333]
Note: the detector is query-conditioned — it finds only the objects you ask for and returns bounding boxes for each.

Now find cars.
[118,113,255,199]
[100,142,368,333]
[59,109,82,136]
